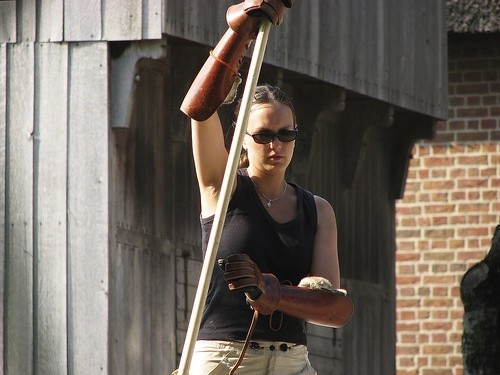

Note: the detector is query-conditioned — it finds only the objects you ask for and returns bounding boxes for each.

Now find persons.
[179,0,351,375]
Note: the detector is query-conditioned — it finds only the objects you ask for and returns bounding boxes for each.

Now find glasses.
[246,120,298,144]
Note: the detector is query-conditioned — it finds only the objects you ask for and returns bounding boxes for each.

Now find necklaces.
[251,179,288,209]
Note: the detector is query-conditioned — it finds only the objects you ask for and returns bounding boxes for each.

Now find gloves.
[180,0,294,122]
[217,252,353,328]
